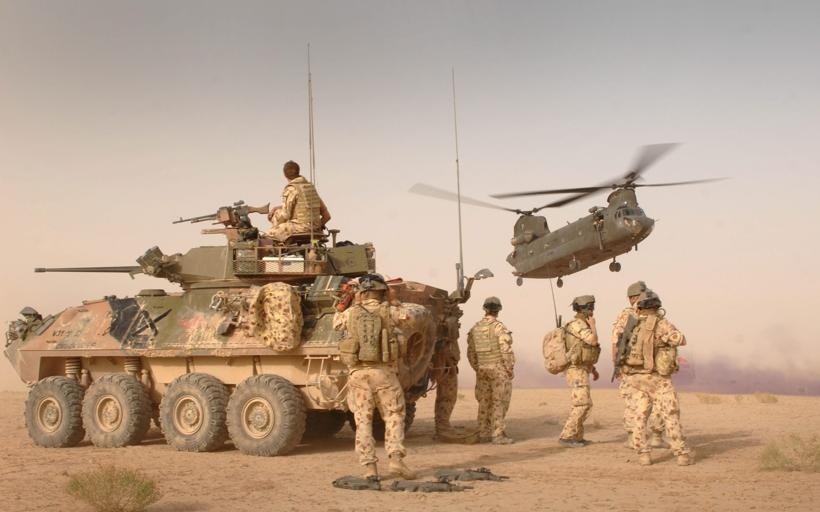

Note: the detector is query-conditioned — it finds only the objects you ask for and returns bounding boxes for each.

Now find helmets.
[572,296,594,307]
[627,282,646,297]
[357,273,388,293]
[482,297,502,308]
[637,290,662,310]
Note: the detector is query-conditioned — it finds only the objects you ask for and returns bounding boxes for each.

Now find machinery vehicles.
[3,38,492,456]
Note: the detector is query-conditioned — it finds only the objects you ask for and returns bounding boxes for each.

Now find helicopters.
[407,141,729,288]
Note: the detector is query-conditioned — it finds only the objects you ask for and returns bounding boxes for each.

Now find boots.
[623,431,695,465]
[387,450,416,480]
[359,463,378,482]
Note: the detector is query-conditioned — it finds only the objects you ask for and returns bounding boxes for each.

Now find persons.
[332,273,417,480]
[436,307,463,437]
[624,291,695,466]
[612,281,670,448]
[467,297,517,444]
[262,161,332,243]
[559,295,601,446]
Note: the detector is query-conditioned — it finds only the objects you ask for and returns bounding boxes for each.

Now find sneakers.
[558,438,592,448]
[491,431,515,445]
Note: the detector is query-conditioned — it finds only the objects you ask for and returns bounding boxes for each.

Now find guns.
[173,201,270,223]
[334,468,510,490]
[611,314,640,384]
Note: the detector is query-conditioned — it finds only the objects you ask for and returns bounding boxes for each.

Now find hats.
[284,161,299,180]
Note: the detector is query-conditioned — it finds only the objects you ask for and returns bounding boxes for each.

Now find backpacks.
[543,319,590,375]
